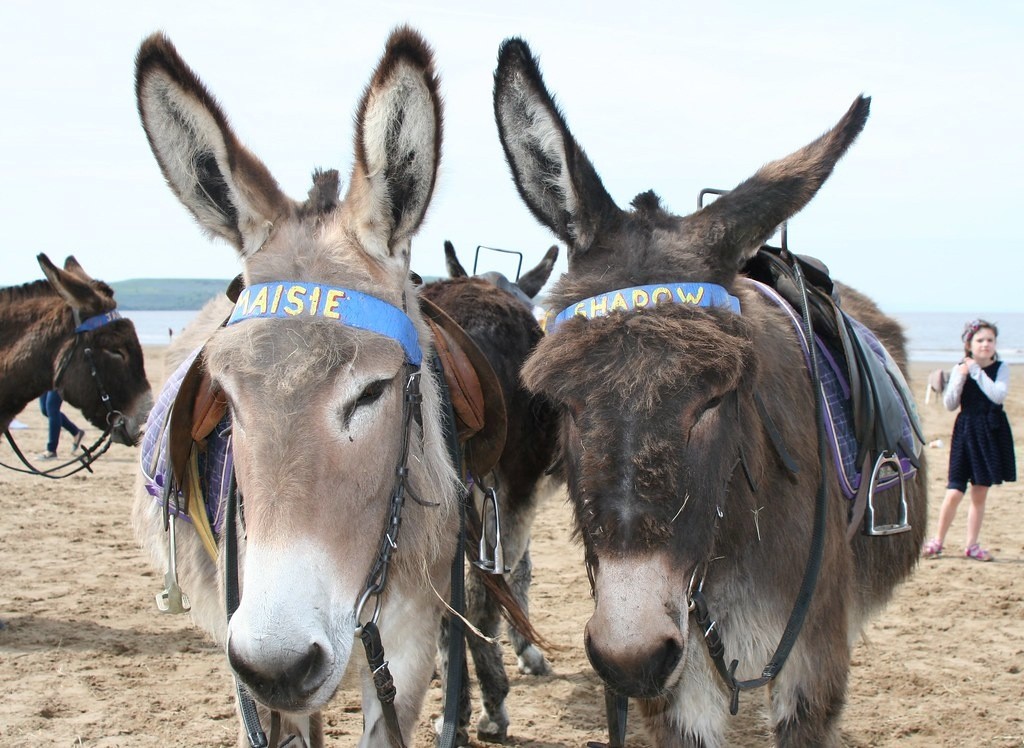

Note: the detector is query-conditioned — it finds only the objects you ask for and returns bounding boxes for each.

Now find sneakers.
[917,538,943,559]
[965,542,993,562]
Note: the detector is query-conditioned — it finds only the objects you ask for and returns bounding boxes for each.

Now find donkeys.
[128,24,561,747]
[493,35,931,748]
[0,251,155,447]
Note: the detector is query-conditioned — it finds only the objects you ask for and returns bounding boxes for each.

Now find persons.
[35,390,84,462]
[921,316,1017,561]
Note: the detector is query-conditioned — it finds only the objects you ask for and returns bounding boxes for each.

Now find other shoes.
[72,430,84,451]
[36,453,56,459]
[9,419,30,429]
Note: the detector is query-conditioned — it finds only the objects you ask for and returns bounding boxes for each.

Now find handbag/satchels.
[926,369,952,403]
